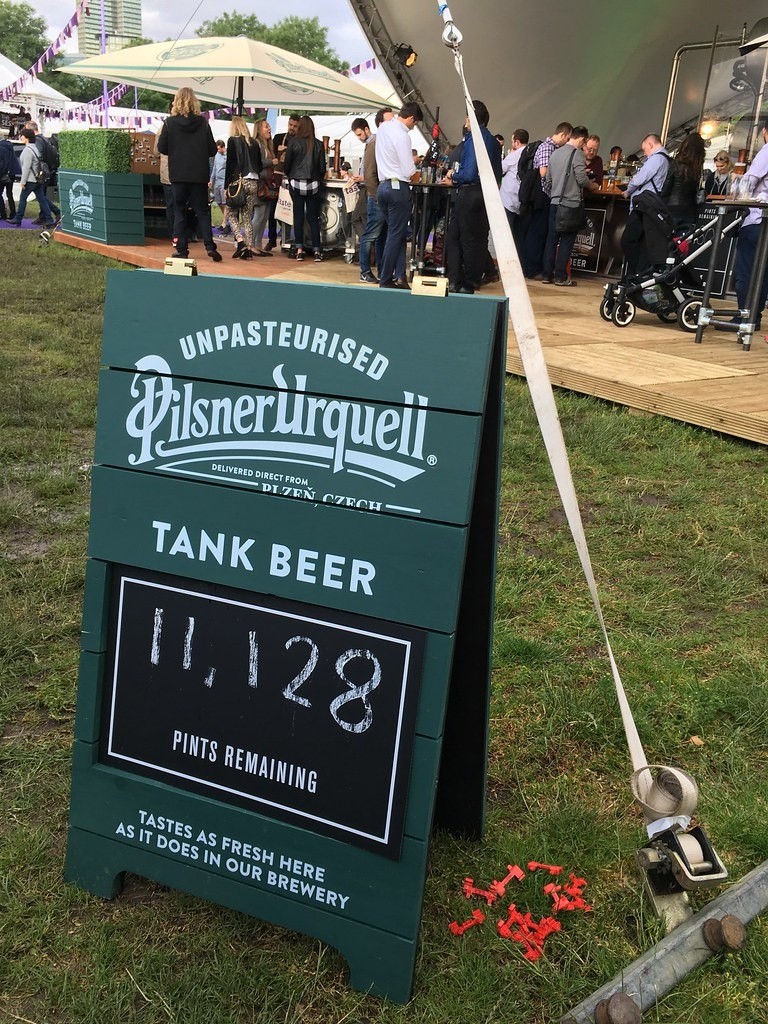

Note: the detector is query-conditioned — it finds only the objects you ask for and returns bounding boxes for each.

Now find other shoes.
[170,223,501,294]
[32,218,43,225]
[715,316,760,332]
[10,213,16,219]
[55,214,60,223]
[0,215,6,220]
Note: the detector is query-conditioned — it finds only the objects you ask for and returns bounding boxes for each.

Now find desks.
[695,200,768,351]
[409,183,454,283]
[281,177,356,264]
[585,189,623,265]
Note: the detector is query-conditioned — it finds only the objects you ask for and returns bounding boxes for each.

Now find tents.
[0,52,71,134]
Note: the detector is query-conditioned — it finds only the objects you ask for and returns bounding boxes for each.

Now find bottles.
[410,161,457,185]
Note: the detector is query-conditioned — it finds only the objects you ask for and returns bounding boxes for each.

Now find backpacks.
[518,140,544,181]
[35,135,58,172]
[26,146,50,185]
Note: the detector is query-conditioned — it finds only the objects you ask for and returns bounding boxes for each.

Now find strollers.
[600,206,751,333]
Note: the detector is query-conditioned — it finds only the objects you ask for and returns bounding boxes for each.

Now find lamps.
[393,43,418,68]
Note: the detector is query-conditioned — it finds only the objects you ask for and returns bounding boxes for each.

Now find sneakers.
[555,276,577,286]
[542,276,552,283]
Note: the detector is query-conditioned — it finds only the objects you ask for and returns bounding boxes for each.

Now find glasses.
[583,139,587,146]
[714,157,725,161]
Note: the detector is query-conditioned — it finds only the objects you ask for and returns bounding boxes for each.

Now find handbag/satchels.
[0,175,11,185]
[342,178,360,214]
[226,182,246,208]
[274,186,294,225]
[258,174,279,202]
[554,204,591,235]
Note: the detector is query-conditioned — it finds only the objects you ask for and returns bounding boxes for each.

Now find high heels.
[7,219,21,226]
[43,221,57,229]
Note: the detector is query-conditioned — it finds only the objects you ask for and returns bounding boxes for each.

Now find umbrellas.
[55,34,401,117]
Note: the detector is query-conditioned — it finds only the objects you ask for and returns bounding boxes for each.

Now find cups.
[602,176,633,192]
[327,168,352,180]
[725,172,755,202]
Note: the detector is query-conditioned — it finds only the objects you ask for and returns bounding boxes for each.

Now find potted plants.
[58,130,146,246]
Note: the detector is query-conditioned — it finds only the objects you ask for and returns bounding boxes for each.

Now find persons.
[340,101,423,288]
[621,118,768,330]
[154,87,222,261]
[208,114,326,261]
[441,100,604,294]
[0,118,60,224]
